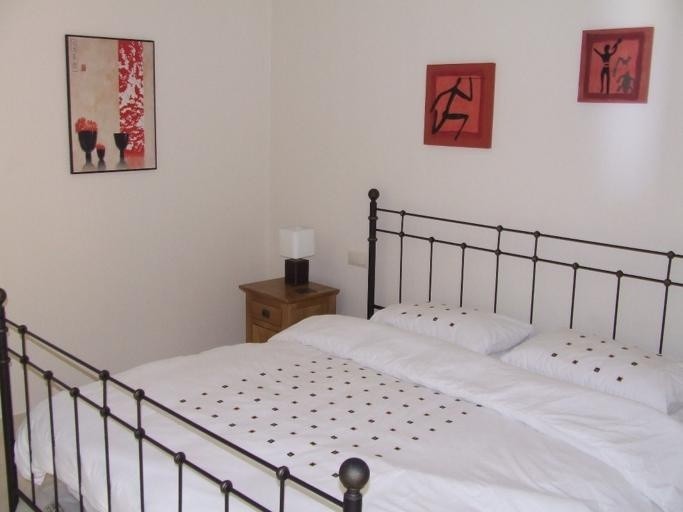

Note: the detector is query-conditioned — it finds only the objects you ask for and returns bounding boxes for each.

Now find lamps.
[278,226,316,286]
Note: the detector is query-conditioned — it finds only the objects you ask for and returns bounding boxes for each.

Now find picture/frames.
[424,63,495,149]
[578,27,655,104]
[64,34,158,175]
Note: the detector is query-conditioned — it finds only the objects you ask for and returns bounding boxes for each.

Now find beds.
[0,188,683,511]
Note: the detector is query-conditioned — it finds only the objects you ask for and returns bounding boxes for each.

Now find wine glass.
[76,130,129,170]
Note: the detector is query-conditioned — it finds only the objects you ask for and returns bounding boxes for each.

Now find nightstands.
[237,278,340,344]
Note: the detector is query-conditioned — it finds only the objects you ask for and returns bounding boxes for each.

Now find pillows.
[499,322,682,416]
[382,299,534,358]
